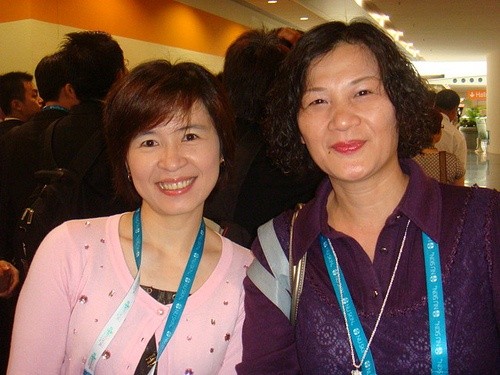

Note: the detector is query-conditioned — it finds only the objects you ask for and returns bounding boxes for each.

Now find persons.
[216,26,309,232]
[412,112,466,191]
[47,27,139,213]
[428,90,467,165]
[237,18,500,374]
[1,243,23,306]
[1,66,44,199]
[7,59,255,375]
[13,53,83,197]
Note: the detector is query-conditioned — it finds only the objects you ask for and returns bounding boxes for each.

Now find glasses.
[436,124,444,129]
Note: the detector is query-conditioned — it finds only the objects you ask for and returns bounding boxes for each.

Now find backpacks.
[11,118,121,281]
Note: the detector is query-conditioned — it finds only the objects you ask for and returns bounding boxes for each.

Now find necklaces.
[323,220,412,375]
